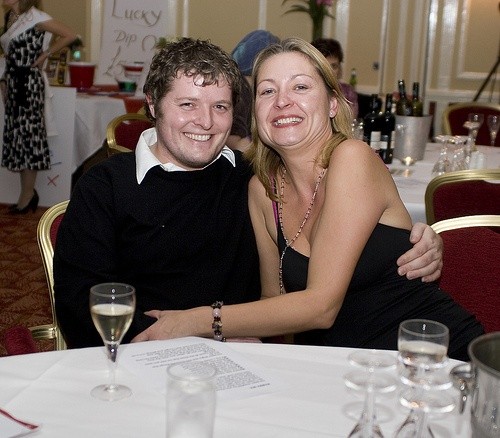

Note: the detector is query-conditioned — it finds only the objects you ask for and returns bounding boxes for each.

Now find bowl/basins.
[119,80,137,93]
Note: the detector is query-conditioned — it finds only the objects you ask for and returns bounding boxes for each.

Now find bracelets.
[211,301,226,343]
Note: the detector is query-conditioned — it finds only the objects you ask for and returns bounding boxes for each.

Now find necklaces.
[278,169,325,297]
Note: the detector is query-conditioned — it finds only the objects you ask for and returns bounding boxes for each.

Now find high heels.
[8,188,38,210]
[8,193,40,215]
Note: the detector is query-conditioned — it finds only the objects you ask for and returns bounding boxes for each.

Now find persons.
[130,36,485,361]
[310,37,359,119]
[52,36,443,351]
[226,29,282,149]
[0,0,80,214]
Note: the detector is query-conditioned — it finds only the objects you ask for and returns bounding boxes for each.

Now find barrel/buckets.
[68,60,97,88]
[392,113,433,163]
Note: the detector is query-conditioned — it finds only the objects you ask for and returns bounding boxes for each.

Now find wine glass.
[342,347,457,438]
[91,283,136,400]
[432,112,500,177]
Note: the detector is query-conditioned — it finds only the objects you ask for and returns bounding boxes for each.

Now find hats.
[230,29,280,76]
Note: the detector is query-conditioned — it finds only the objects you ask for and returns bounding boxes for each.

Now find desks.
[0,87,146,208]
[0,342,500,438]
[366,140,500,226]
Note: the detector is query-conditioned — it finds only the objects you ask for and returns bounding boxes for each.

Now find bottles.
[362,79,423,164]
[350,67,358,87]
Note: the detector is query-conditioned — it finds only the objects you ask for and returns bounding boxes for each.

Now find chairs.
[29,107,154,354]
[425,102,500,334]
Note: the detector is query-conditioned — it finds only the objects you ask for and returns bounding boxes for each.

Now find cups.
[167,361,218,438]
[399,319,449,368]
[452,331,500,438]
